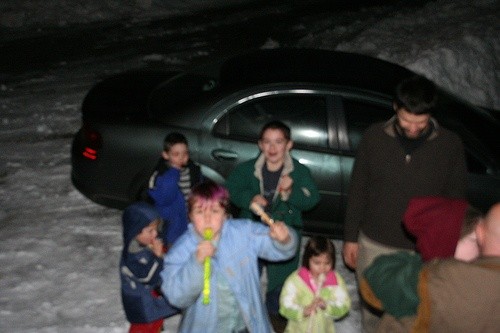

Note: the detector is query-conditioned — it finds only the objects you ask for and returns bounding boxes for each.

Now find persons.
[340,84,474,333]
[117,199,182,333]
[145,132,209,256]
[278,233,350,333]
[221,119,318,333]
[158,180,299,333]
[367,203,500,333]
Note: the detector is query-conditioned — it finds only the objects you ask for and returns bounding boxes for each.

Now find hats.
[403,197,469,262]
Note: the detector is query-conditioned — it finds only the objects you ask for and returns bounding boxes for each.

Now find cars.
[69,47,500,245]
